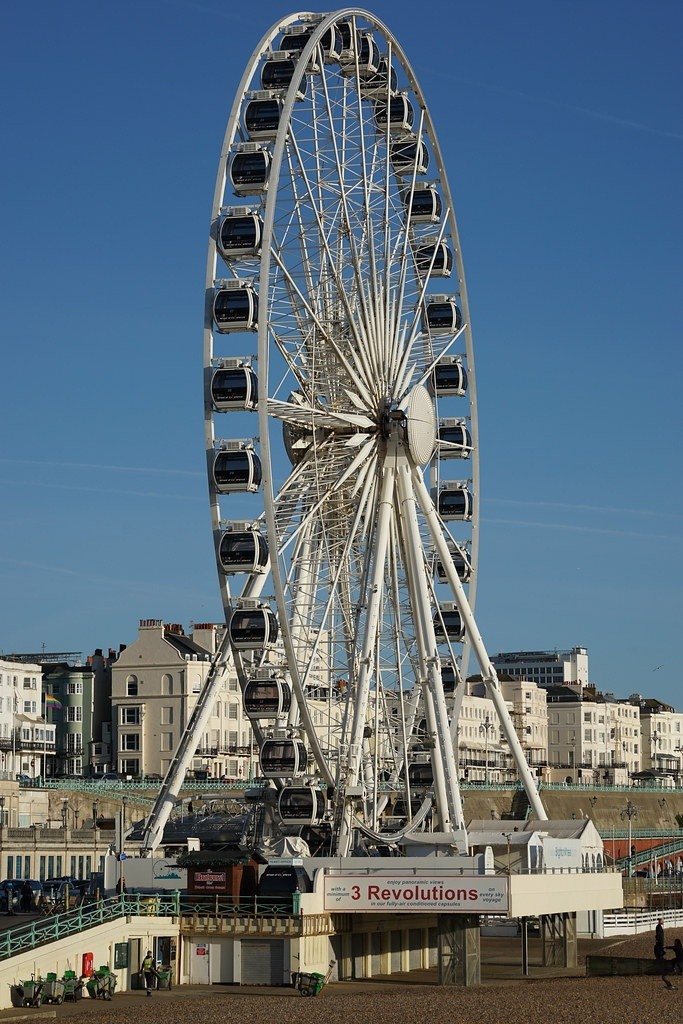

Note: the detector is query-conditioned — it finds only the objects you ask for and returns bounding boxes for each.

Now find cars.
[16,774,31,784]
[0,875,91,912]
[55,774,86,784]
[88,771,118,783]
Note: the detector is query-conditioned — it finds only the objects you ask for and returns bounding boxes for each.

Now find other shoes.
[147,991,152,997]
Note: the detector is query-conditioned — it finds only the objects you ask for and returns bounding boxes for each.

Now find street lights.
[619,800,639,877]
[90,802,99,829]
[122,796,129,824]
[62,801,69,828]
[478,715,495,787]
[500,832,513,874]
[0,797,6,827]
[74,810,81,830]
[570,736,577,784]
[647,730,662,772]
[61,808,65,827]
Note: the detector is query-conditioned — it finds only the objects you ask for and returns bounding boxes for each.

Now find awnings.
[47,695,63,708]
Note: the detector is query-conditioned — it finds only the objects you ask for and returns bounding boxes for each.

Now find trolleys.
[155,964,174,991]
[11,968,84,1010]
[293,971,333,998]
[83,971,119,1001]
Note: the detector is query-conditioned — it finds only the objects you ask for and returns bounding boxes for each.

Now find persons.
[116,877,128,915]
[663,939,683,975]
[653,918,666,975]
[5,880,33,913]
[139,951,156,997]
[657,860,680,879]
